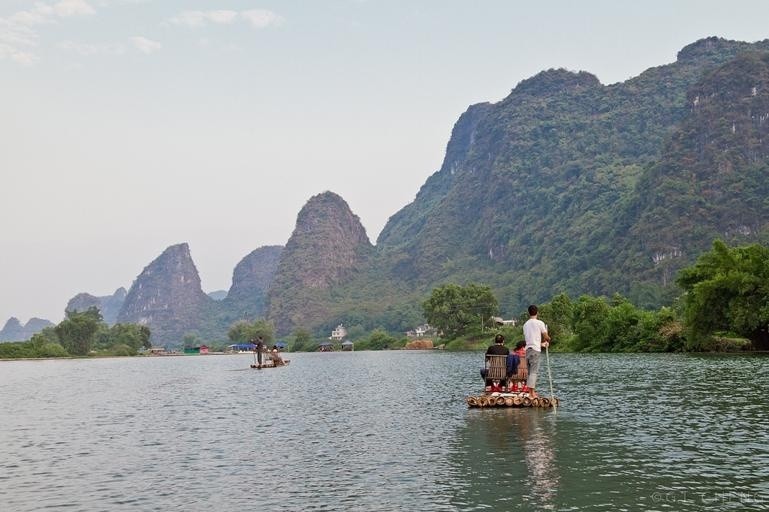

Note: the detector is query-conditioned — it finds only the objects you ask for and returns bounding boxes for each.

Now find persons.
[480,335,509,391]
[522,305,550,398]
[251,336,263,366]
[513,339,528,392]
[272,345,288,366]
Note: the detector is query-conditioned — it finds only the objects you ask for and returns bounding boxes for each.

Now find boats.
[467,381,563,412]
[250,358,292,368]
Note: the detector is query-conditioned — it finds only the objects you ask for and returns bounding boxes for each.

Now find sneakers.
[491,384,527,392]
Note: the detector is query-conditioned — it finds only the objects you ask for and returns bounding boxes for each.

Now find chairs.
[509,356,528,385]
[485,354,508,392]
